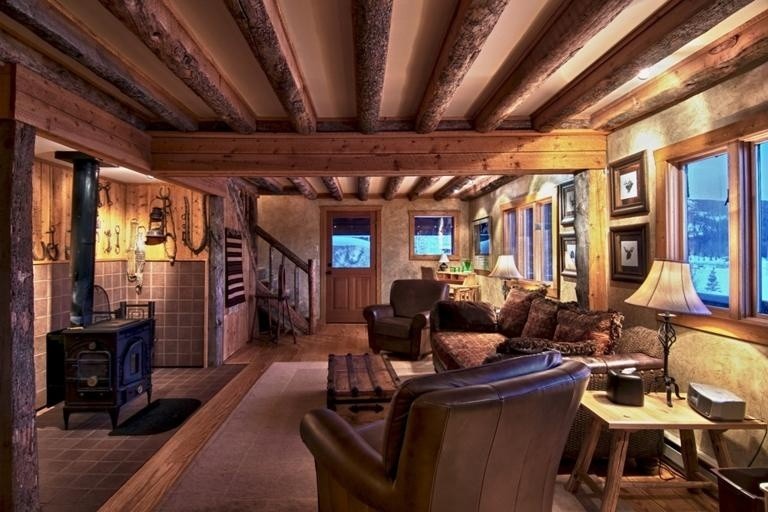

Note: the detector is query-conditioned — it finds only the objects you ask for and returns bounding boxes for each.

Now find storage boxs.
[709,467,768,512]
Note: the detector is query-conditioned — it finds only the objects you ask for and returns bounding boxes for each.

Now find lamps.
[144,188,171,238]
[439,254,449,270]
[489,254,521,299]
[626,259,712,407]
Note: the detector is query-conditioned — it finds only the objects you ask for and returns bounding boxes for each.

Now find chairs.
[363,278,450,360]
[300,350,592,512]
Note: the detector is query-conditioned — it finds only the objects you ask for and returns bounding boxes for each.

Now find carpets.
[35,364,247,512]
[151,360,437,512]
[108,398,201,435]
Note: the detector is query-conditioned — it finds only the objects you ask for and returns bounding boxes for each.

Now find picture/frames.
[607,150,649,217]
[610,223,651,283]
[559,233,577,278]
[557,178,576,225]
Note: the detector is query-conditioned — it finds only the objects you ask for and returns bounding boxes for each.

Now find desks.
[436,270,477,302]
[246,294,296,346]
[62,301,156,429]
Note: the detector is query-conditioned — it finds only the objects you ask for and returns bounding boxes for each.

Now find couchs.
[431,299,666,467]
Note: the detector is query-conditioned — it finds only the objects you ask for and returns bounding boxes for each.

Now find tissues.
[608,367,644,406]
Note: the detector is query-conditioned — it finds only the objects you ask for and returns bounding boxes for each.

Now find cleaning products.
[437,248,451,271]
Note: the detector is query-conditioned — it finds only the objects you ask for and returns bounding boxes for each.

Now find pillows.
[498,288,625,358]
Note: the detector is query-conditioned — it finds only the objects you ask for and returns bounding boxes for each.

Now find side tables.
[565,388,768,512]
